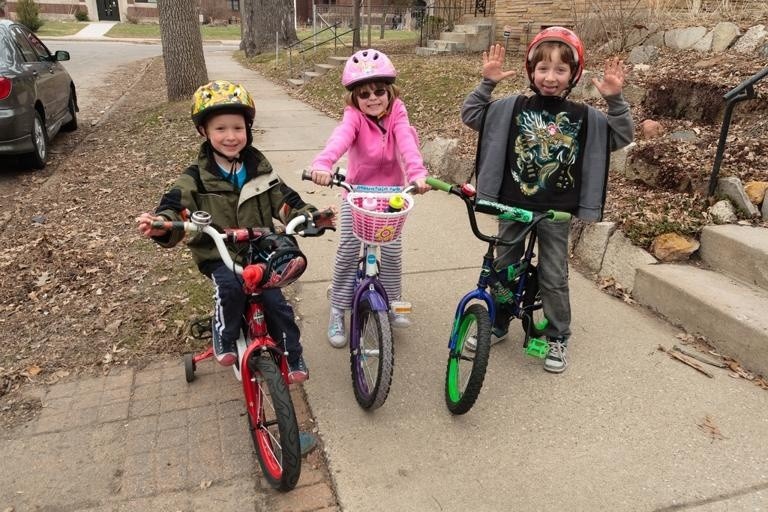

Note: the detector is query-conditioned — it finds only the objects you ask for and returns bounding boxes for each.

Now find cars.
[0,19,81,168]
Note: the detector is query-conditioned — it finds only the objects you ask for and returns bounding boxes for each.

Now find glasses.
[356,88,388,100]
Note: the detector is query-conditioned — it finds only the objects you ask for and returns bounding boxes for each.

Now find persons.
[308,49,433,349]
[457,26,635,374]
[135,81,336,383]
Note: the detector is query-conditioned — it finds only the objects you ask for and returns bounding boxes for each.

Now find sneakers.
[328,305,348,349]
[463,326,511,353]
[388,308,411,329]
[210,314,239,367]
[543,336,569,374]
[285,351,310,383]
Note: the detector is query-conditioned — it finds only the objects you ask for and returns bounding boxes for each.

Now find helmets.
[189,79,256,137]
[524,25,585,90]
[340,48,397,91]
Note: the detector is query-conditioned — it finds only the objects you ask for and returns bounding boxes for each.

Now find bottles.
[388,195,405,213]
[361,196,379,212]
[241,262,270,289]
